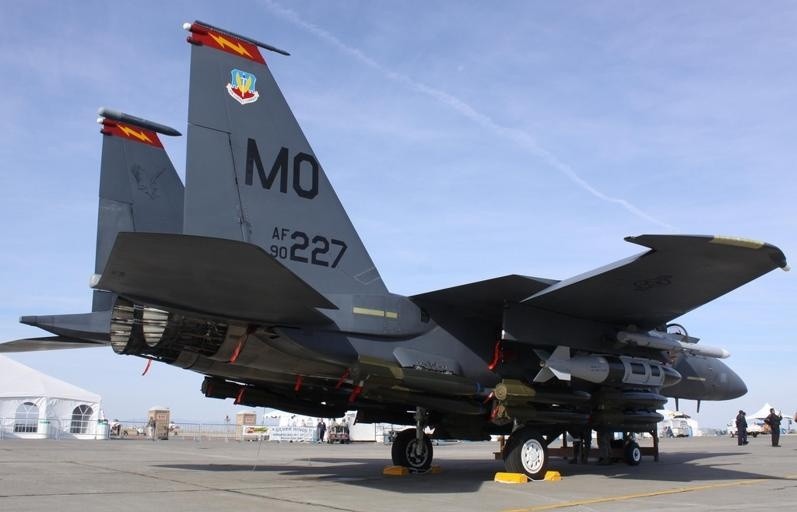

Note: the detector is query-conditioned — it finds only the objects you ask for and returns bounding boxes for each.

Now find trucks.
[727,419,764,438]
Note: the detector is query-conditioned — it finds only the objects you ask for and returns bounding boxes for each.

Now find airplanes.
[1,20,789,479]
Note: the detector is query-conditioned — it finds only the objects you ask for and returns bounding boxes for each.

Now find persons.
[764,408,782,447]
[794,413,797,423]
[736,410,744,445]
[742,412,749,444]
[667,426,673,438]
[146,417,156,440]
[318,418,349,444]
